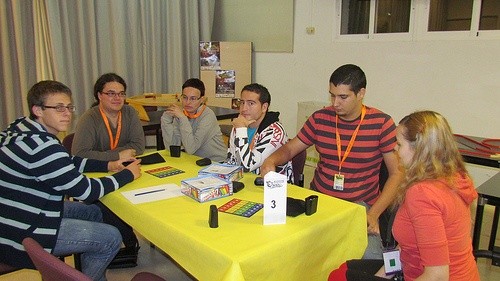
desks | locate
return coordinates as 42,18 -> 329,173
449,134 -> 500,267
76,148 -> 368,281
82,103 -> 241,151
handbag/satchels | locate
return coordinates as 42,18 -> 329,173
96,200 -> 141,269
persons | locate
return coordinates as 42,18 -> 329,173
71,72 -> 146,161
161,78 -> 228,164
260,64 -> 403,259
0,80 -> 142,281
327,110 -> 480,281
227,83 -> 294,184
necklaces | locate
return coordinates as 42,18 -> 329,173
333,104 -> 366,191
182,102 -> 206,119
250,134 -> 257,151
97,105 -> 121,151
382,204 -> 402,274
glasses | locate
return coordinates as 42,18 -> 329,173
102,90 -> 127,97
180,94 -> 201,101
44,104 -> 76,112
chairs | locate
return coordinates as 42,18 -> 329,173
23,237 -> 169,281
292,149 -> 306,186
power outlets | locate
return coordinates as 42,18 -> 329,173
307,27 -> 315,34
